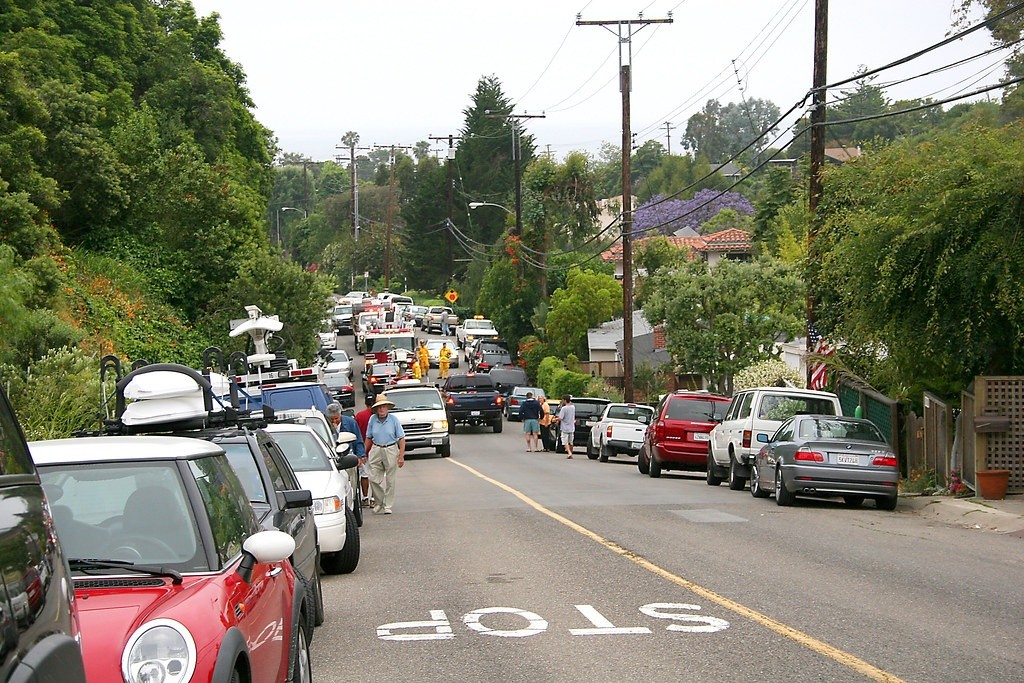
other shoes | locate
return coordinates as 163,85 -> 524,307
526,449 -> 530,452
567,455 -> 573,459
372,505 -> 383,513
370,499 -> 376,507
361,496 -> 369,507
383,507 -> 392,514
535,449 -> 538,452
540,448 -> 550,452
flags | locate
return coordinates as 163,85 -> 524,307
805,321 -> 835,390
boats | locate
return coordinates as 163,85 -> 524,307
100,346 -> 247,436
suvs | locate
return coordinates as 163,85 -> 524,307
380,381 -> 451,459
706,386 -> 843,490
638,390 -> 732,478
549,395 -> 612,451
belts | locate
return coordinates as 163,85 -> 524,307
373,443 -> 395,448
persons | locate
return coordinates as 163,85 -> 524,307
437,343 -> 453,379
324,403 -> 367,498
364,394 -> 406,514
440,308 -> 452,337
537,395 -> 551,452
353,393 -> 376,508
417,339 -> 432,376
519,392 -> 545,453
554,395 -> 576,459
412,357 -> 422,380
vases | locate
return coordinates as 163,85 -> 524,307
976,469 -> 1010,500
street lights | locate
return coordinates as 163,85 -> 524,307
469,203 -> 522,289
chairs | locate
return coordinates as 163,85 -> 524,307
277,438 -> 304,462
123,487 -> 195,560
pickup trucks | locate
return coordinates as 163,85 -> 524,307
442,374 -> 504,434
585,400 -> 654,461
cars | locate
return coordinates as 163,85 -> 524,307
25,435 -> 314,683
324,348 -> 354,379
219,289 -> 561,427
0,384 -> 87,683
750,414 -> 899,511
322,372 -> 356,407
262,423 -> 360,575
180,424 -> 324,625
274,404 -> 364,527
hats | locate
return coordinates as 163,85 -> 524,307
371,394 -> 395,410
